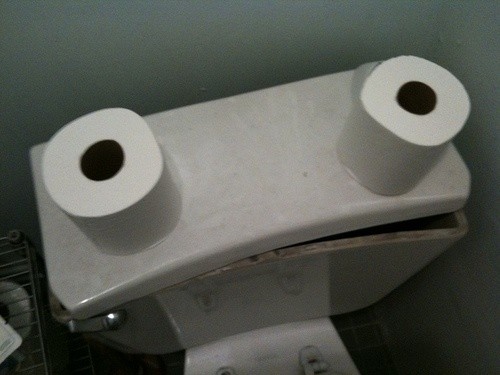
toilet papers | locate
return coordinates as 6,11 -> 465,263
1,281 -> 33,341
334,55 -> 472,196
41,107 -> 183,256
